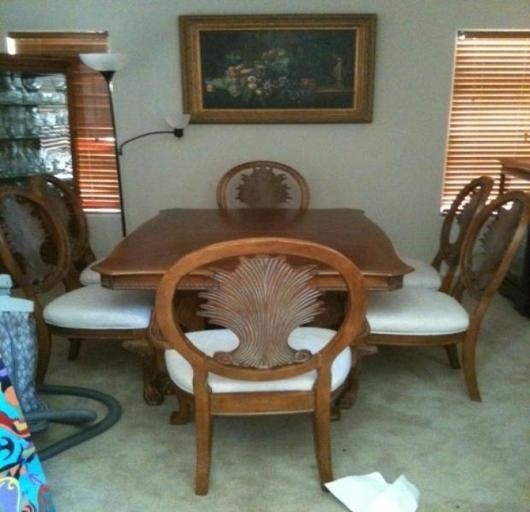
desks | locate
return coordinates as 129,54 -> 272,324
91,207 -> 414,404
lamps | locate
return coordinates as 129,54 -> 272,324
78,52 -> 191,239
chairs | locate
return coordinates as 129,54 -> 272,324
155,237 -> 368,494
339,175 -> 529,411
215,163 -> 310,212
4,172 -> 164,407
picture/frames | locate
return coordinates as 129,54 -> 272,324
178,14 -> 377,125
